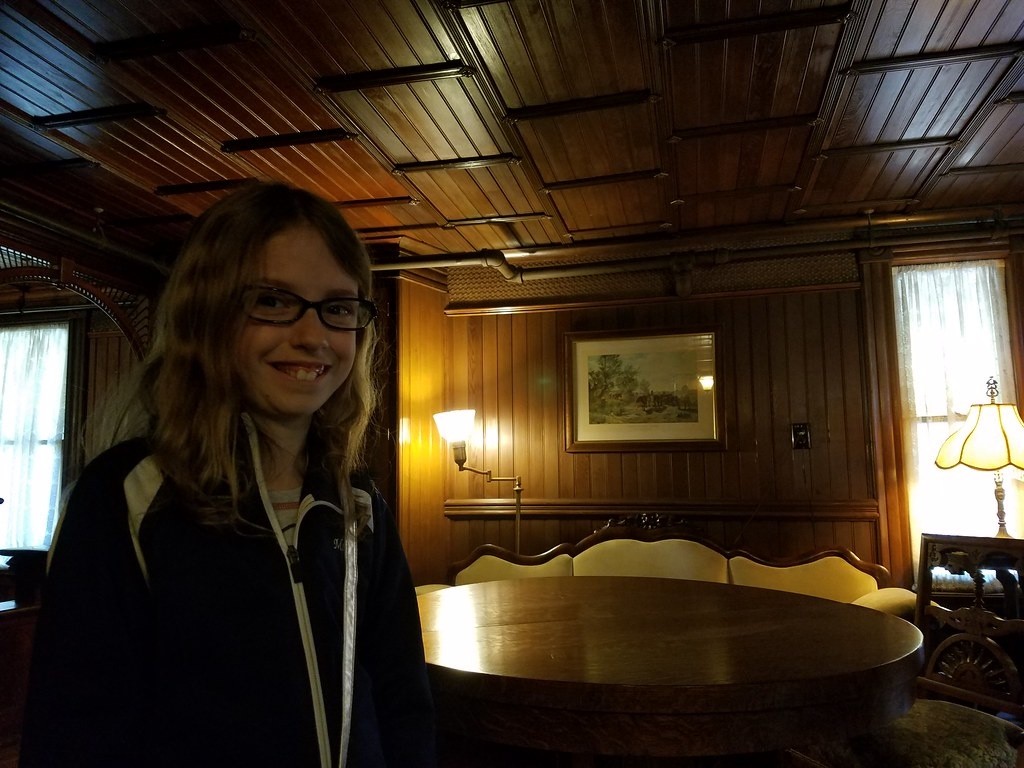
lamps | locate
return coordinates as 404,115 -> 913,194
435,408 -> 522,556
935,378 -> 1024,538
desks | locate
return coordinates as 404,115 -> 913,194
419,578 -> 1024,768
910,534 -> 1024,725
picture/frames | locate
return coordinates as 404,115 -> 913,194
563,327 -> 722,453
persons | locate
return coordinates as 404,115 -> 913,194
16,181 -> 439,768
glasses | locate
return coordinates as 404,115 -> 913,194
240,285 -> 379,331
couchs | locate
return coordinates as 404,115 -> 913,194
451,511 -> 917,615
